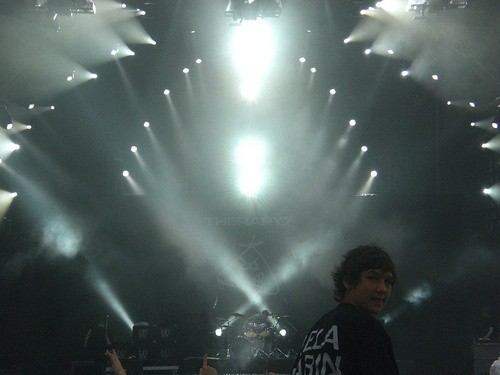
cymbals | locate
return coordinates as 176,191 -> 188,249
231,312 -> 243,319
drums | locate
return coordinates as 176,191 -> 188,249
244,323 -> 257,338
227,336 -> 253,359
257,322 -> 271,337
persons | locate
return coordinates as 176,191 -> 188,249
290,245 -> 400,375
83,306 -> 500,375
